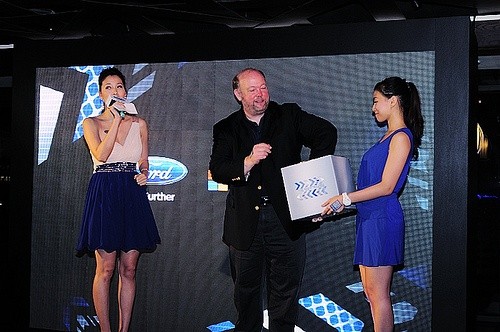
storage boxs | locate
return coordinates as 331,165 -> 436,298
280,155 -> 357,221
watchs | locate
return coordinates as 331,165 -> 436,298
342,192 -> 352,207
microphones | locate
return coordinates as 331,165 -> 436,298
107,95 -> 126,117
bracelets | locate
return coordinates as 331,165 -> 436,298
140,167 -> 149,172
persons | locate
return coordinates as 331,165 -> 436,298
76,68 -> 162,332
311,76 -> 425,332
207,67 -> 339,332
